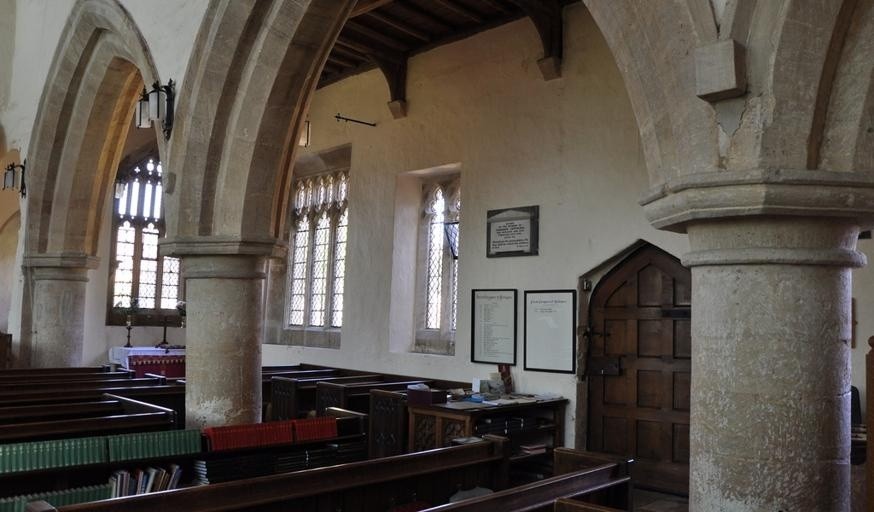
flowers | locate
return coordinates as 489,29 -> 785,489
176,301 -> 186,316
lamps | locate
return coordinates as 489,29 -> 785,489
135,77 -> 176,141
2,158 -> 27,199
299,119 -> 312,146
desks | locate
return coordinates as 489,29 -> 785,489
0,346 -> 636,512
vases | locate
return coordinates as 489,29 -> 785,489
181,316 -> 186,328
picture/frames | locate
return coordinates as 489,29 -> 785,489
470,288 -> 517,366
486,205 -> 540,258
523,289 -> 577,374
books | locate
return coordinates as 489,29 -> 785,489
475,391 -> 564,455
0,414 -> 338,512
